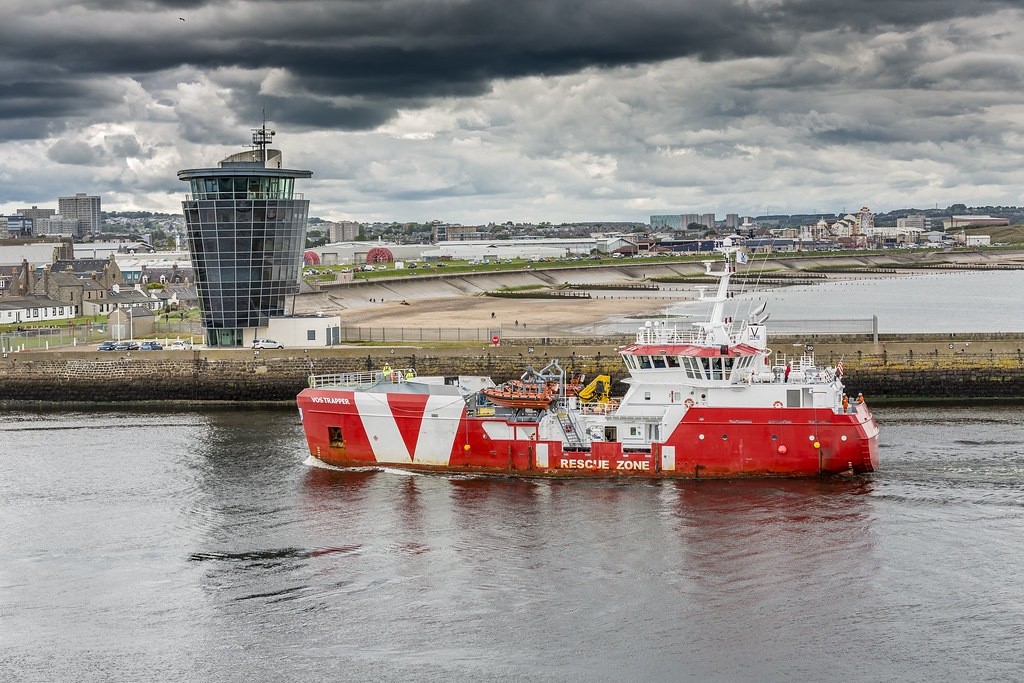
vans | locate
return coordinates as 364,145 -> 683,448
613,253 -> 622,259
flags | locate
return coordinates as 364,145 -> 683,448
838,361 -> 843,379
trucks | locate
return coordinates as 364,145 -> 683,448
395,262 -> 405,269
530,255 -> 540,261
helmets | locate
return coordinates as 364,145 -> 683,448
385,363 -> 388,365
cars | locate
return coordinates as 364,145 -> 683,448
408,263 -> 418,268
550,256 -> 583,262
469,259 -> 478,265
115,341 -> 139,350
524,259 -> 533,263
423,263 -> 430,268
378,265 -> 386,270
437,262 -> 448,267
322,269 -> 332,274
171,341 -> 192,351
309,268 -> 321,275
97,341 -> 117,351
504,260 -> 512,264
586,255 -> 602,260
538,258 -> 550,262
139,340 -> 164,350
364,265 -> 374,271
251,337 -> 285,349
629,251 -> 691,258
494,259 -> 501,265
303,271 -> 312,275
341,267 -> 352,272
481,259 -> 489,265
355,267 -> 362,272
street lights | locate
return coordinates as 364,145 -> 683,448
126,310 -> 132,340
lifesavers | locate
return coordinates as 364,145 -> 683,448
684,399 -> 695,408
609,400 -> 618,409
773,401 -> 784,409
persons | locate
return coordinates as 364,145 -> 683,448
857,393 -> 864,404
784,363 -> 791,383
406,369 -> 413,379
842,392 -> 848,413
383,363 -> 391,380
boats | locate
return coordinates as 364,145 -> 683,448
295,238 -> 886,480
482,356 -> 585,409
